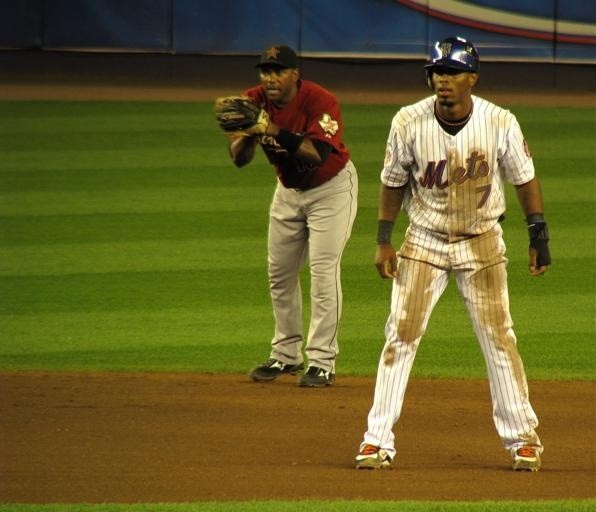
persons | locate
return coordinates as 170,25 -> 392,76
356,35 -> 552,473
214,45 -> 358,388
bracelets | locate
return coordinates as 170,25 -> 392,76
275,129 -> 304,155
527,213 -> 548,240
376,220 -> 394,244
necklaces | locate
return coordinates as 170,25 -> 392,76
435,110 -> 472,126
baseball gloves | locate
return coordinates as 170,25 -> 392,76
215,94 -> 270,136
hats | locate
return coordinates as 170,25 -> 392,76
253,45 -> 297,67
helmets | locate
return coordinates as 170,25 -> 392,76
424,36 -> 479,91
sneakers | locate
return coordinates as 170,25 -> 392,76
354,445 -> 391,469
296,366 -> 334,387
249,358 -> 304,381
511,448 -> 541,472
